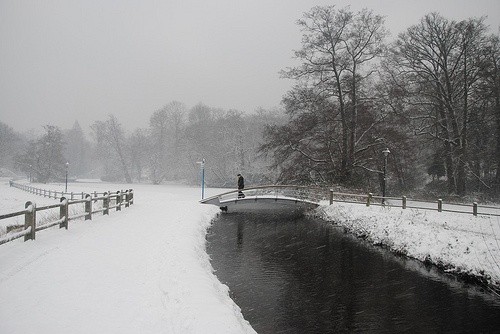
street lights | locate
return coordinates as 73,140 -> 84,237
30,164 -> 32,183
65,162 -> 68,192
382,148 -> 391,204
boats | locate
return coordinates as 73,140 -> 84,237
199,158 -> 322,213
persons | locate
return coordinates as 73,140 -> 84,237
236,173 -> 245,199
